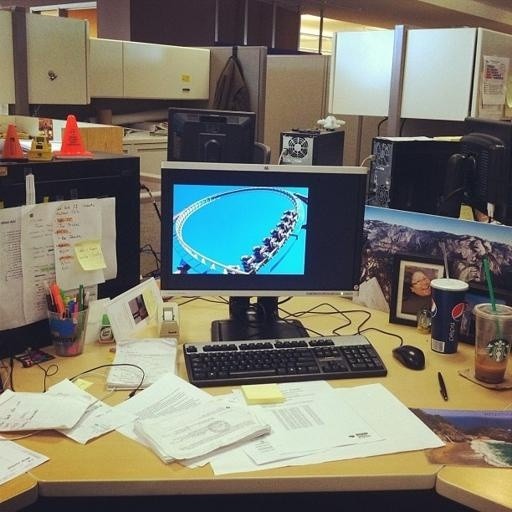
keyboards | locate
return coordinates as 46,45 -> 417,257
184,336 -> 386,386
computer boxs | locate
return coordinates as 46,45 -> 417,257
0,152 -> 140,359
368,136 -> 463,214
280,129 -> 344,166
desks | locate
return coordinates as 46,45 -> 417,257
0,290 -> 512,512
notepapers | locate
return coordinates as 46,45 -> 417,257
241,384 -> 284,405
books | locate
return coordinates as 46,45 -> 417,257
136,395 -> 272,465
105,337 -> 180,391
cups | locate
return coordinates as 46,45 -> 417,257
47,308 -> 90,356
95,108 -> 113,125
427,278 -> 471,352
473,303 -> 512,383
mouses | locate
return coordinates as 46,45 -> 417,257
392,345 -> 425,370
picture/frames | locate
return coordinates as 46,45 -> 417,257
388,253 -> 446,328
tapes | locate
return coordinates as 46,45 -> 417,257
164,311 -> 172,321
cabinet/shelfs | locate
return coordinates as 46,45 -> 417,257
121,136 -> 168,176
0,13 -> 90,106
89,36 -> 211,101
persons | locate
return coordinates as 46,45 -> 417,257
402,270 -> 431,316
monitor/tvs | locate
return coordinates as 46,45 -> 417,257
167,107 -> 256,164
441,118 -> 512,226
159,161 -> 367,340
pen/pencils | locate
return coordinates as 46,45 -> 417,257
438,372 -> 448,400
42,280 -> 90,342
